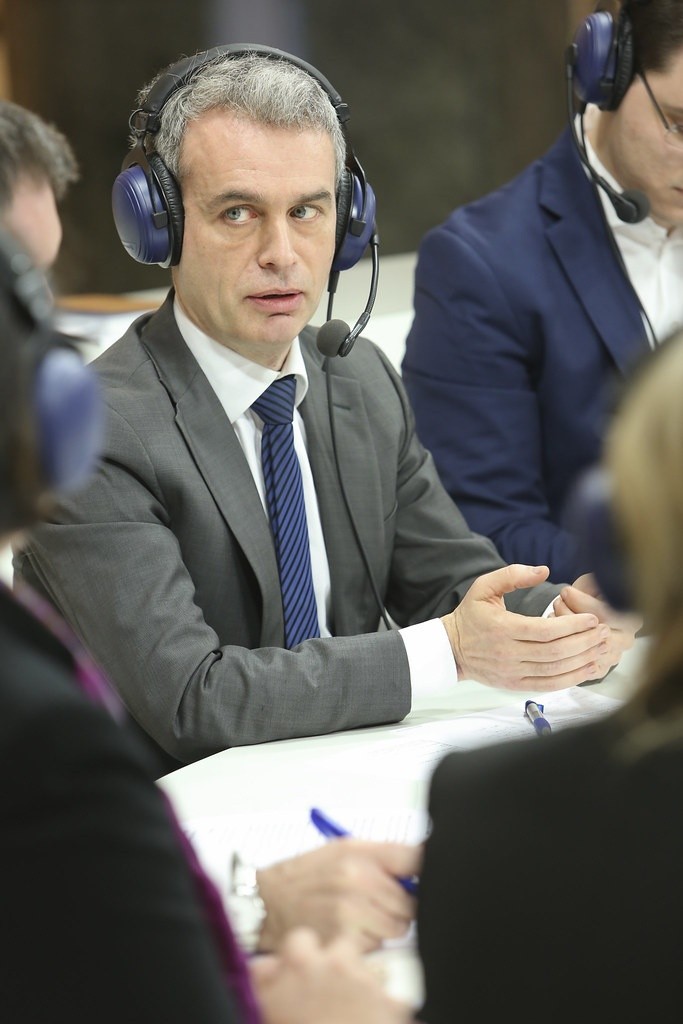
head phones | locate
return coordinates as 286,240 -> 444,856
111,43 -> 376,271
1,221 -> 107,495
565,0 -> 635,113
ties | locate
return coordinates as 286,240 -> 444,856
12,575 -> 266,1024
250,378 -> 321,650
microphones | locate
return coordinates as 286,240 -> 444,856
565,77 -> 651,223
316,245 -> 379,357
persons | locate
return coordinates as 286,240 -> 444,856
14,42 -> 644,782
411,324 -> 683,1024
0,97 -> 426,1024
399,0 -> 683,601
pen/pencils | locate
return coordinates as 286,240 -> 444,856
308,807 -> 420,898
525,699 -> 551,737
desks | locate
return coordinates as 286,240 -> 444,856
153,637 -> 650,1008
